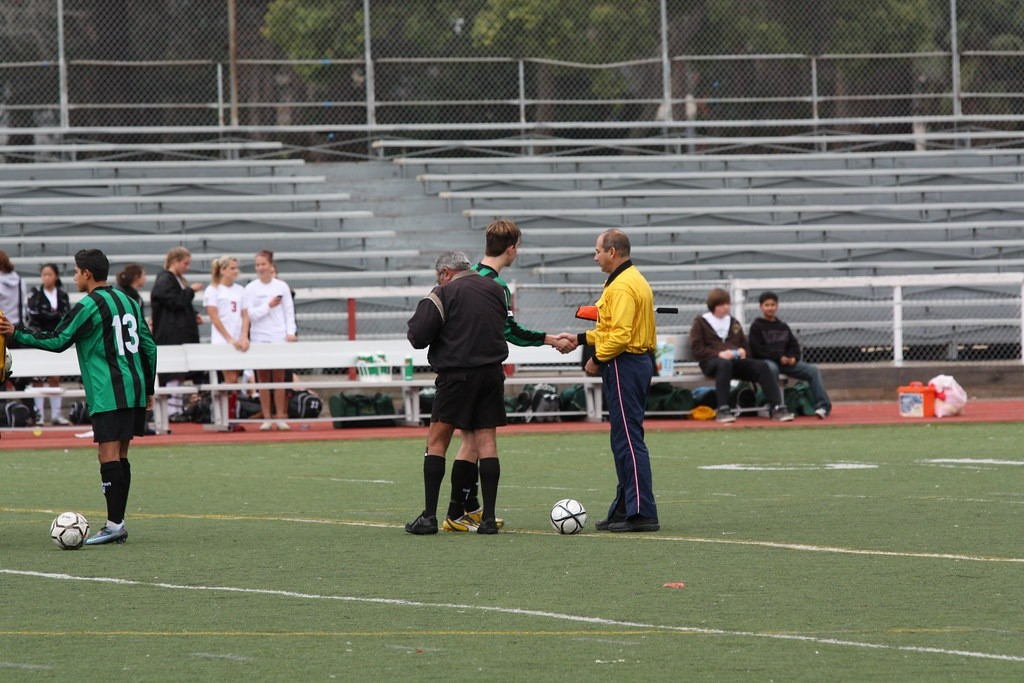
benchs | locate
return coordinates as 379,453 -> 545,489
0,130 -> 1024,347
0,386 -> 199,435
195,368 -> 797,433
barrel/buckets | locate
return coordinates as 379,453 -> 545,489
897,382 -> 935,418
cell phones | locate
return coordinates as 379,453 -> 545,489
277,295 -> 282,298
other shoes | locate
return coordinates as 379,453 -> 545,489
771,405 -> 795,422
51,415 -> 69,425
716,408 -> 736,422
260,421 -> 272,429
814,408 -> 826,419
84,523 -> 128,544
273,419 -> 290,431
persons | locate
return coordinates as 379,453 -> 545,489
689,288 -> 793,423
0,249 -> 157,546
443,217 -> 576,532
552,229 -> 660,533
406,250 -> 509,535
749,292 -> 831,419
115,247 -> 204,436
202,251 -> 298,433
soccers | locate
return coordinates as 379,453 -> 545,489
49,511 -> 90,550
550,499 -> 588,534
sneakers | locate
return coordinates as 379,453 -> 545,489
477,517 -> 498,534
442,514 -> 480,532
596,515 -> 628,530
404,511 -> 438,534
608,516 -> 660,532
460,510 -> 504,528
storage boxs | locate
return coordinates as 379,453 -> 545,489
897,381 -> 936,418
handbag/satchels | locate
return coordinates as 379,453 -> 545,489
2,382 -> 827,420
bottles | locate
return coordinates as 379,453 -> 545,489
405,354 -> 413,380
358,352 -> 386,376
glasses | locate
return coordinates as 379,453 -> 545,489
435,271 -> 444,286
